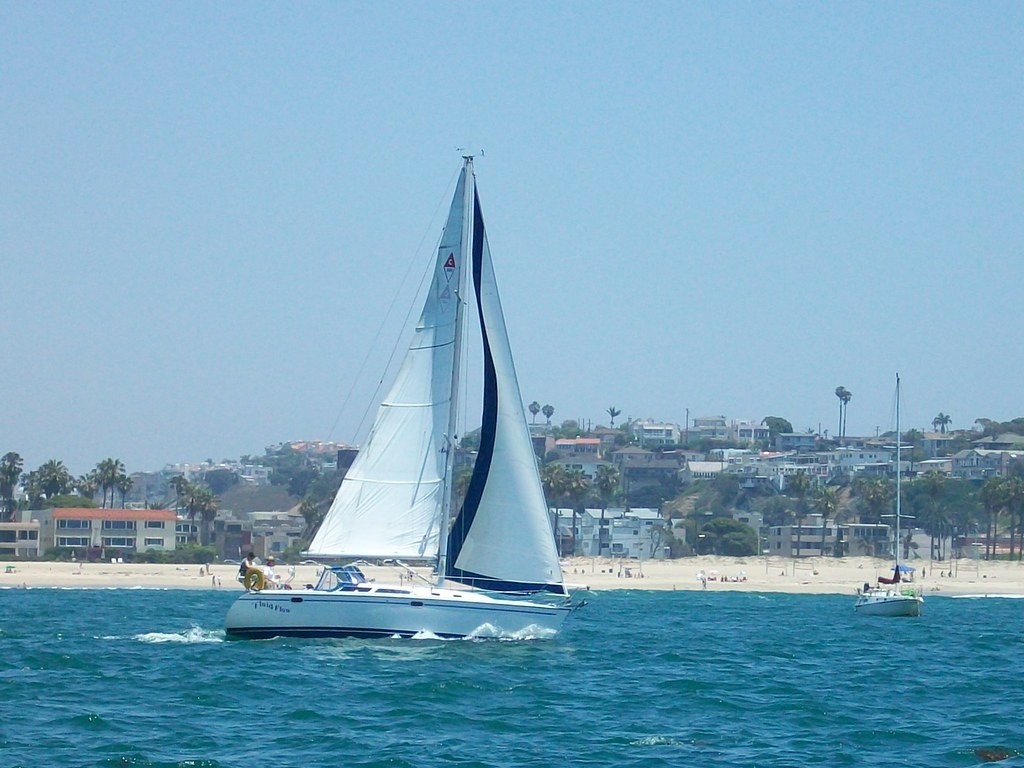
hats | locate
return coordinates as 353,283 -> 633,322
247,552 -> 256,558
266,555 -> 275,561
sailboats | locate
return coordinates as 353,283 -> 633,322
853,374 -> 925,618
221,143 -> 594,643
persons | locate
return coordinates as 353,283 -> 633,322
200,561 -> 222,588
241,551 -> 282,588
